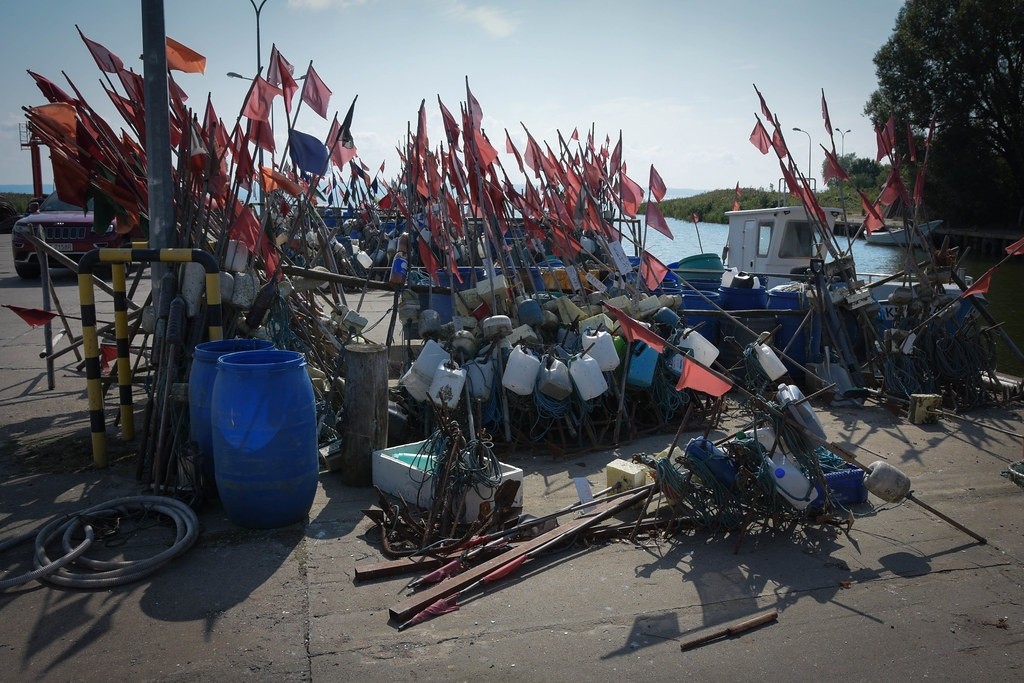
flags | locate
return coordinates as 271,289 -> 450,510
2,34 -> 1024,399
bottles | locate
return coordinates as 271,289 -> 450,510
355,251 -> 375,267
579,235 -> 594,255
393,258 -> 825,510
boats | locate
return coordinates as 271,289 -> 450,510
863,220 -> 944,247
717,176 -> 991,342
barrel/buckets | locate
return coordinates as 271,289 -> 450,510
187,338 -> 282,454
680,287 -> 717,360
718,284 -> 774,388
766,290 -> 822,384
210,350 -> 320,530
402,234 -> 727,325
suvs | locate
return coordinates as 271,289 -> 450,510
11,190 -> 152,280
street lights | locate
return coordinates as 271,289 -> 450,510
792,127 -> 811,189
836,128 -> 851,221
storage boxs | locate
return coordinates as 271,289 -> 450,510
746,428 -> 868,511
371,440 -> 523,523
